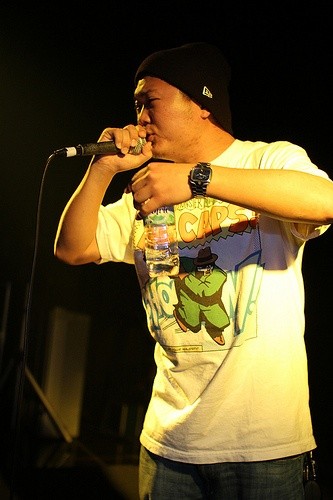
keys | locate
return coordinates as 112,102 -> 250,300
303,449 -> 319,486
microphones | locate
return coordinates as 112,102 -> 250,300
55,136 -> 147,159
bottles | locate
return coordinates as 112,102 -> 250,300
142,196 -> 180,277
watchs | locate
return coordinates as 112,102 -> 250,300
189,161 -> 213,199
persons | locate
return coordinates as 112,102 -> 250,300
53,41 -> 332,500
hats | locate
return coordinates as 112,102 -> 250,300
134,40 -> 232,133
